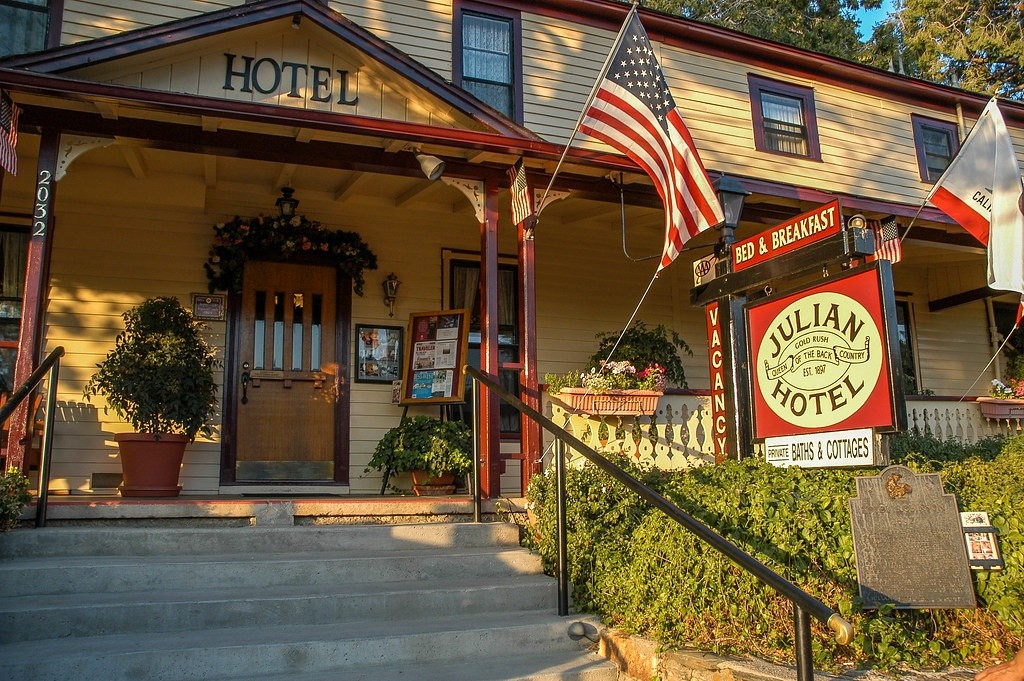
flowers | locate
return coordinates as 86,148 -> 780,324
988,377 -> 1024,400
545,359 -> 669,396
203,211 -> 378,299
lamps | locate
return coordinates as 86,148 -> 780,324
413,145 -> 445,180
382,271 -> 403,319
620,172 -> 751,261
275,185 -> 300,218
848,210 -> 867,230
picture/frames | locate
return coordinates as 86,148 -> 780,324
355,323 -> 403,384
190,292 -> 226,321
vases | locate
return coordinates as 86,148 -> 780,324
558,387 -> 664,416
975,396 -> 1024,430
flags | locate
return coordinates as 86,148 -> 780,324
925,97 -> 1024,329
869,214 -> 901,264
579,6 -> 724,275
0,88 -> 21,176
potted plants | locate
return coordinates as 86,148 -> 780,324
82,295 -> 226,497
359,414 -> 474,496
